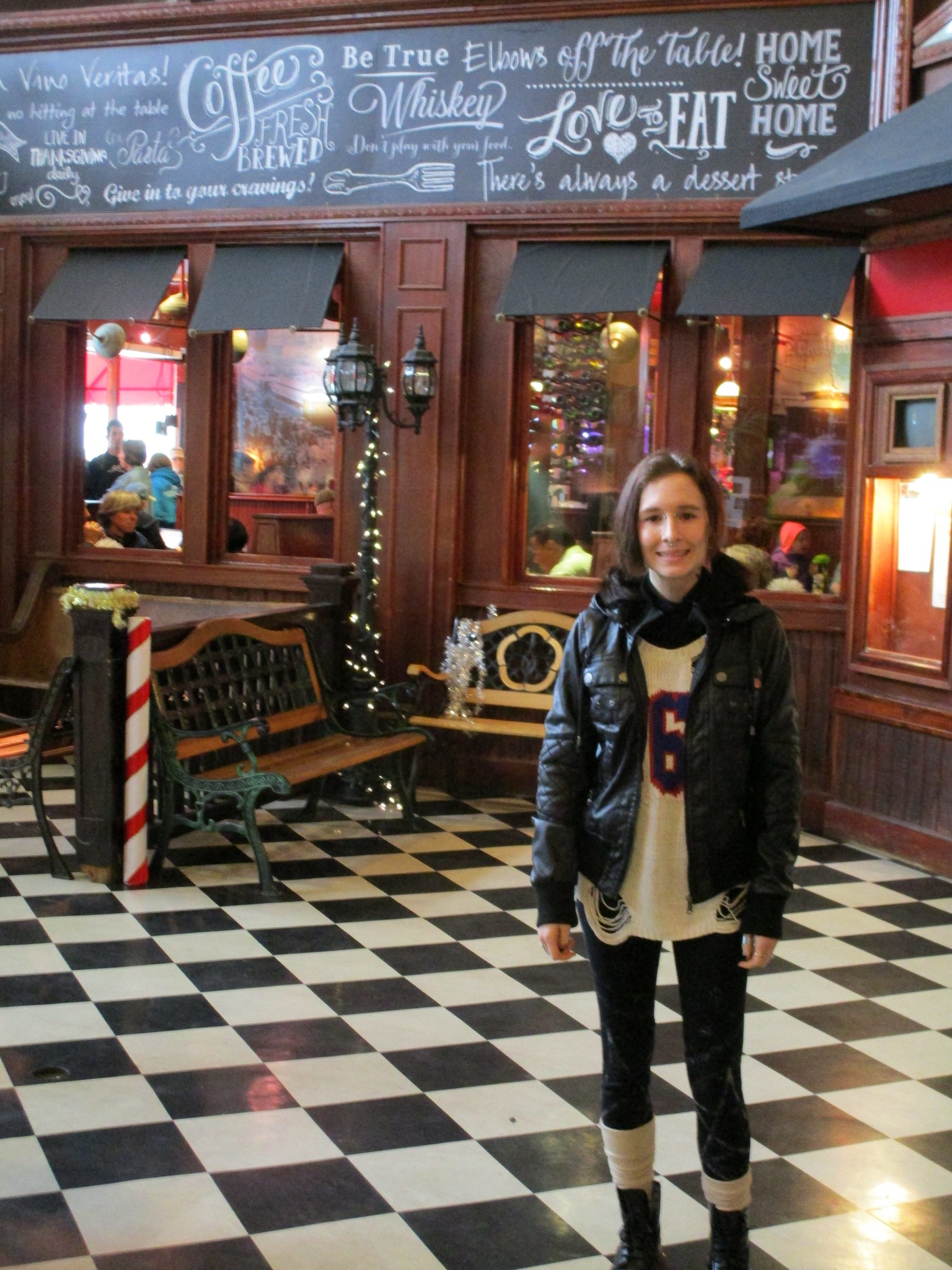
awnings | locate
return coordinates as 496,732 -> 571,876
676,237 -> 864,316
740,84 -> 952,236
493,240 -> 672,313
29,243 -> 186,324
189,238 -> 345,336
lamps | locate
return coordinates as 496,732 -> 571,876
160,259 -> 190,322
85,315 -> 126,357
800,329 -> 850,409
598,312 -> 638,364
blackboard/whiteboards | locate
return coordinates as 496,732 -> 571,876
0,0 -> 913,240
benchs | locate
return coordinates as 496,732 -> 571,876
145,621 -> 433,896
0,655 -> 79,882
407,610 -> 585,808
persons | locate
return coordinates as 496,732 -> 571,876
231,448 -> 328,498
530,450 -> 805,1270
531,519 -> 593,577
724,517 -> 774,590
82,419 -> 185,550
226,515 -> 250,554
314,489 -> 335,518
770,522 -> 814,586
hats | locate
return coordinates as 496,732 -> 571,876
127,482 -> 156,502
171,446 -> 185,460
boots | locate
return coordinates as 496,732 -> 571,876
611,1180 -> 661,1270
708,1202 -> 750,1270
